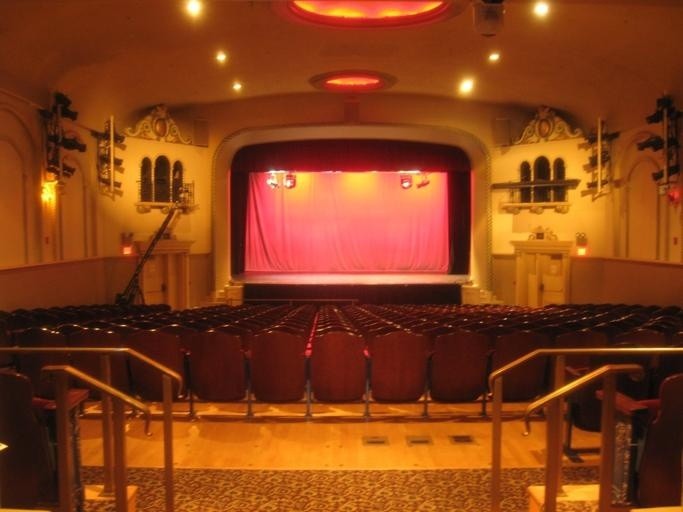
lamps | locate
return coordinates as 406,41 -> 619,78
577,235 -> 587,256
121,233 -> 133,255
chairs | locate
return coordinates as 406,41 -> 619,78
595,376 -> 683,508
0,369 -> 58,509
